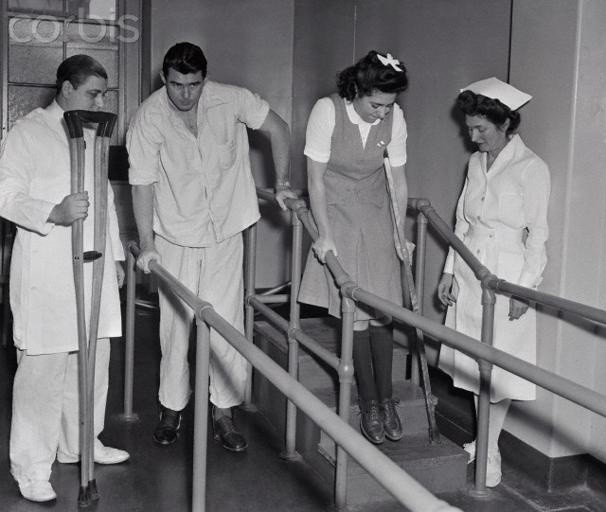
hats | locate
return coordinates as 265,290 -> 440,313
460,76 -> 533,112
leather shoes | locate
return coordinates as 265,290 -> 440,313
154,409 -> 182,445
359,398 -> 404,444
213,415 -> 248,453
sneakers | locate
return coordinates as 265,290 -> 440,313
57,444 -> 130,464
463,441 -> 477,464
9,467 -> 57,503
486,448 -> 502,487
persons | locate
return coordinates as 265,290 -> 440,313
0,55 -> 131,503
438,77 -> 551,488
126,42 -> 299,451
297,50 -> 417,444
